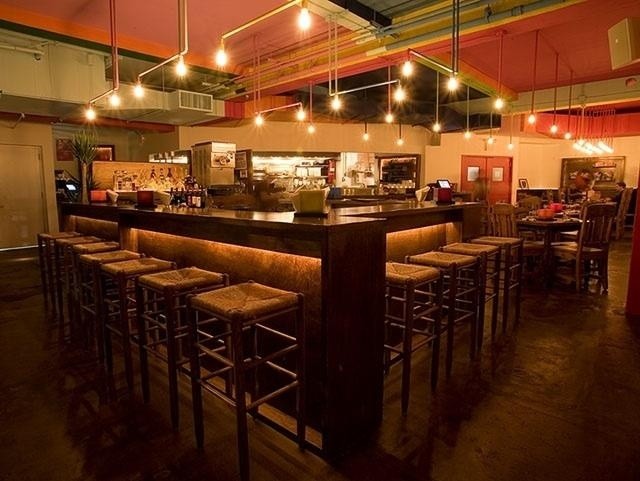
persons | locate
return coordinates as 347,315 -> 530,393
608,181 -> 637,225
575,169 -> 595,193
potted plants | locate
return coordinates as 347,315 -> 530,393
62,131 -> 100,206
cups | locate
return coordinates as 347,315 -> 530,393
562,209 -> 571,222
529,210 -> 537,221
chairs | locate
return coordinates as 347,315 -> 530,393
488,187 -> 633,303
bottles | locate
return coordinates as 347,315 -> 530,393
112,169 -> 140,192
149,167 -> 200,189
170,186 -> 205,209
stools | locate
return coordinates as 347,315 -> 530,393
37,230 -> 120,337
134,266 -> 233,451
78,248 -> 144,375
101,257 -> 182,410
383,236 -> 534,418
185,280 -> 309,480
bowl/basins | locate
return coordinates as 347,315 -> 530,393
550,203 -> 562,212
290,187 -> 330,214
538,208 -> 555,218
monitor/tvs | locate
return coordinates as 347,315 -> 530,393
66,184 -> 76,190
438,180 -> 449,187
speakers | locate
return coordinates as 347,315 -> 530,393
607,18 -> 640,70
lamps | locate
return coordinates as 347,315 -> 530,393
82,0 -> 619,162
